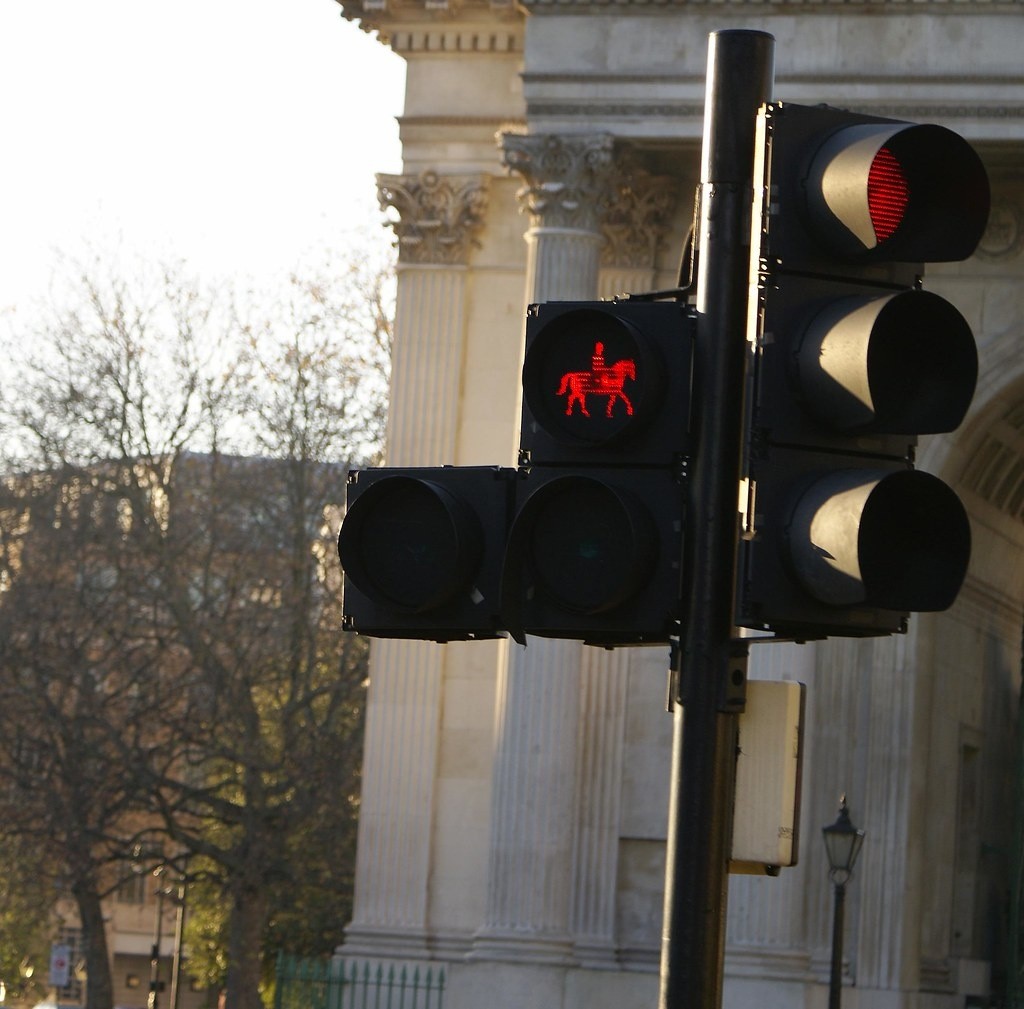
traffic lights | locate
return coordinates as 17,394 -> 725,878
333,297 -> 699,647
724,94 -> 994,639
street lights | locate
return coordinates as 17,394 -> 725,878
819,793 -> 867,1009
